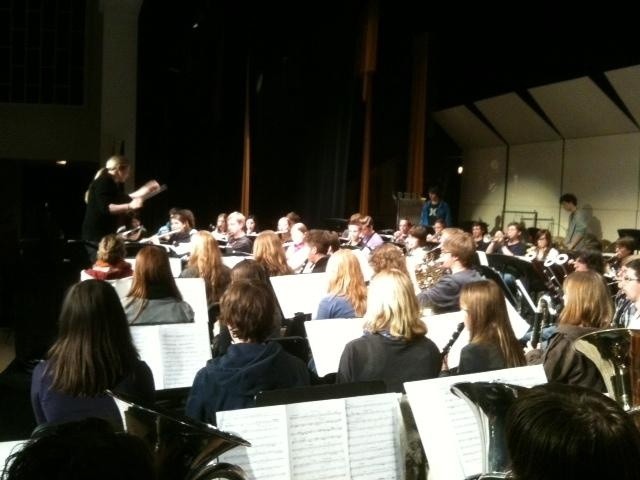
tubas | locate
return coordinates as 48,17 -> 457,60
572,327 -> 640,431
447,379 -> 532,478
103,383 -> 256,480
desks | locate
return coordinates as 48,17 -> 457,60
125,237 -> 255,258
149,369 -> 456,419
397,198 -> 425,230
15,240 -> 83,365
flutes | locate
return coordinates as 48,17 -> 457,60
438,319 -> 465,367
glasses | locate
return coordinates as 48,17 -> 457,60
621,277 -> 639,283
441,250 -> 457,255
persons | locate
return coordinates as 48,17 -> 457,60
279,211 -> 301,243
470,222 -> 488,249
82,234 -> 131,285
523,274 -> 633,392
281,222 -> 311,274
611,237 -> 637,267
507,384 -> 638,479
211,260 -> 286,357
212,213 -> 231,244
120,249 -> 193,325
341,223 -> 363,248
176,230 -> 232,311
221,213 -> 253,258
243,218 -> 255,239
454,281 -> 525,373
249,231 -> 294,276
495,221 -> 532,260
612,260 -> 639,331
86,155 -> 143,258
29,281 -> 155,428
116,211 -> 146,243
420,185 -> 452,228
405,225 -> 438,294
170,212 -> 199,252
558,192 -> 593,249
523,230 -> 558,265
370,244 -> 408,277
575,252 -> 603,277
318,251 -> 367,321
336,269 -> 443,391
419,228 -> 495,315
186,281 -> 311,423
393,218 -> 410,245
299,230 -> 330,274
359,217 -> 380,247
1,416 -> 158,479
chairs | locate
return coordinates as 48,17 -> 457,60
286,314 -> 310,336
268,336 -> 308,364
254,380 -> 388,406
